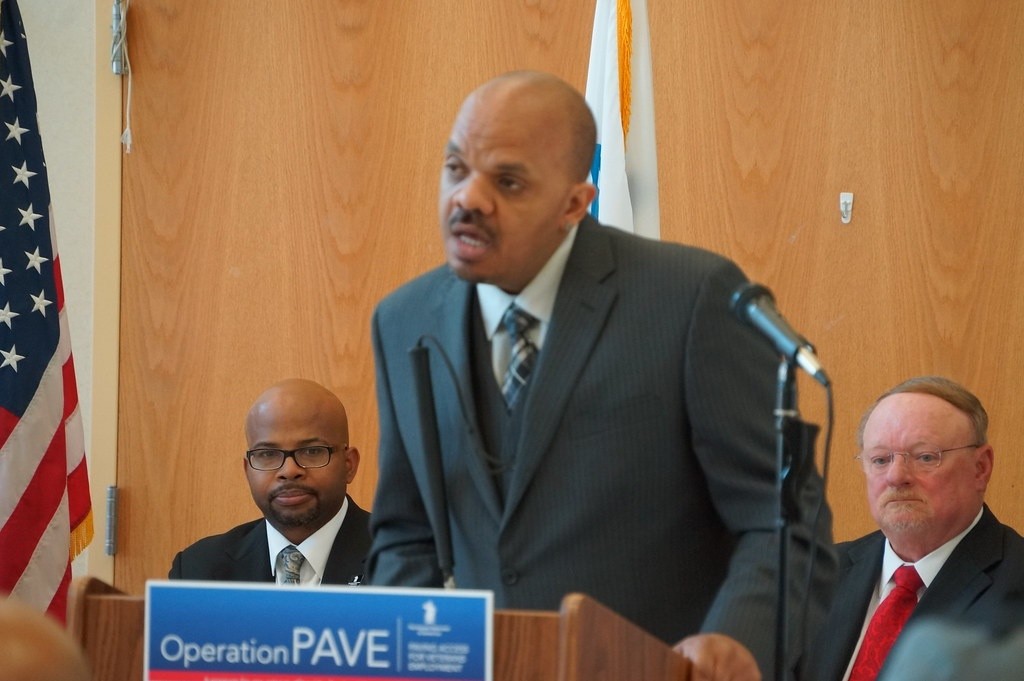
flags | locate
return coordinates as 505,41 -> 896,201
0,0 -> 94,633
584,0 -> 663,242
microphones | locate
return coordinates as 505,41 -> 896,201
728,284 -> 829,391
409,349 -> 455,570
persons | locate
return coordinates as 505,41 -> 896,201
361,70 -> 841,680
832,376 -> 1024,680
166,379 -> 374,586
0,598 -> 91,681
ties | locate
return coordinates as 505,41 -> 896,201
847,566 -> 926,681
500,304 -> 541,415
277,547 -> 306,584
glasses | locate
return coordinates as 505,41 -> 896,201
246,443 -> 350,471
852,443 -> 979,475
